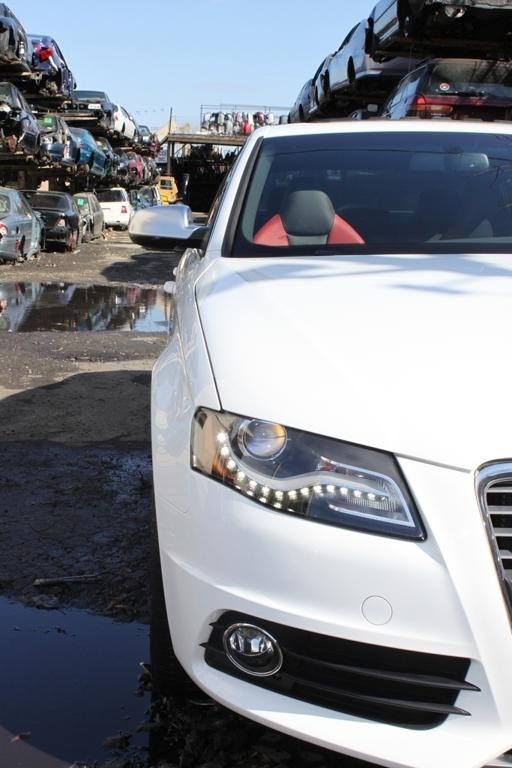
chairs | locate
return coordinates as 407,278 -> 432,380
253,188 -> 367,246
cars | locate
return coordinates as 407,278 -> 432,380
287,1 -> 512,121
128,122 -> 512,759
